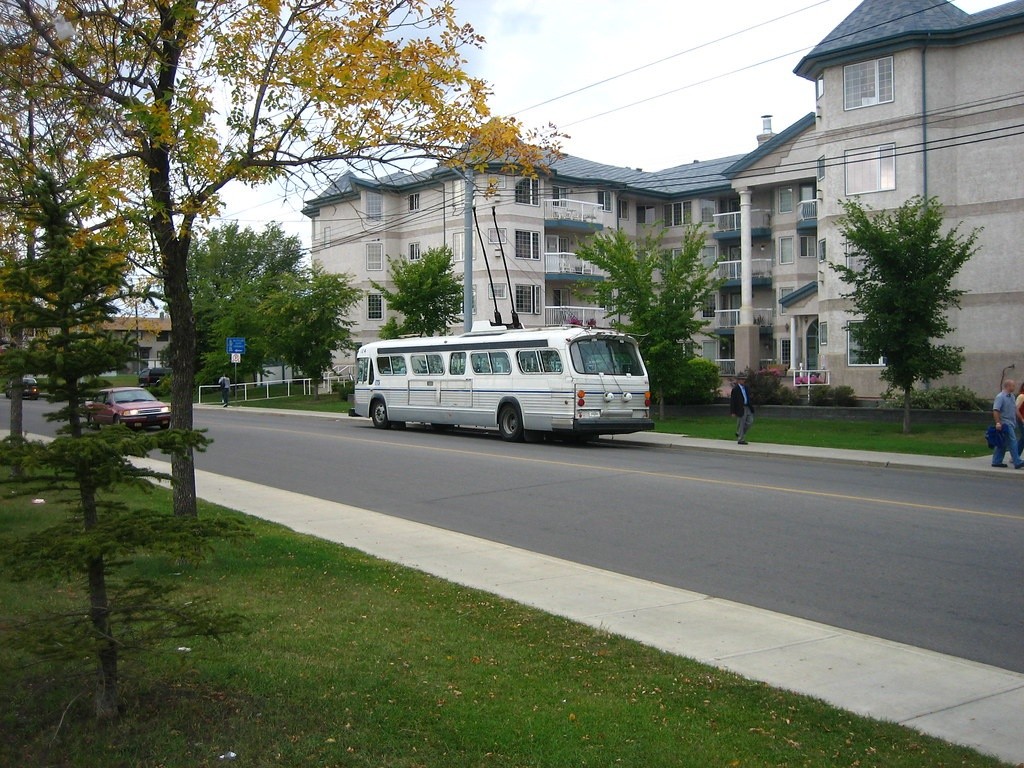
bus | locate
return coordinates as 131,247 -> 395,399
349,324 -> 655,443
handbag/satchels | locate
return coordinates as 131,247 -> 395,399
221,376 -> 225,389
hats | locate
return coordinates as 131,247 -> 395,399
738,373 -> 747,378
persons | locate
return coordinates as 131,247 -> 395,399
991,379 -> 1024,469
218,373 -> 230,407
731,372 -> 755,445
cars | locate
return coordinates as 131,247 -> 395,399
138,367 -> 172,387
5,377 -> 39,400
86,387 -> 173,432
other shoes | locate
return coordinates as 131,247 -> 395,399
1015,461 -> 1024,469
736,432 -> 739,437
992,463 -> 1007,467
738,442 -> 748,444
223,404 -> 227,407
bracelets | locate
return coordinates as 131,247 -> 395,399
995,422 -> 1001,424
